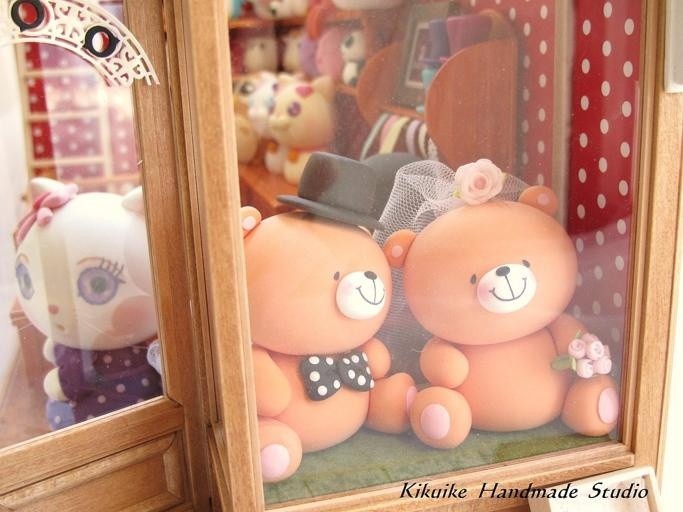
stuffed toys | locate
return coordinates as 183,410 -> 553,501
9,0 -> 625,485
